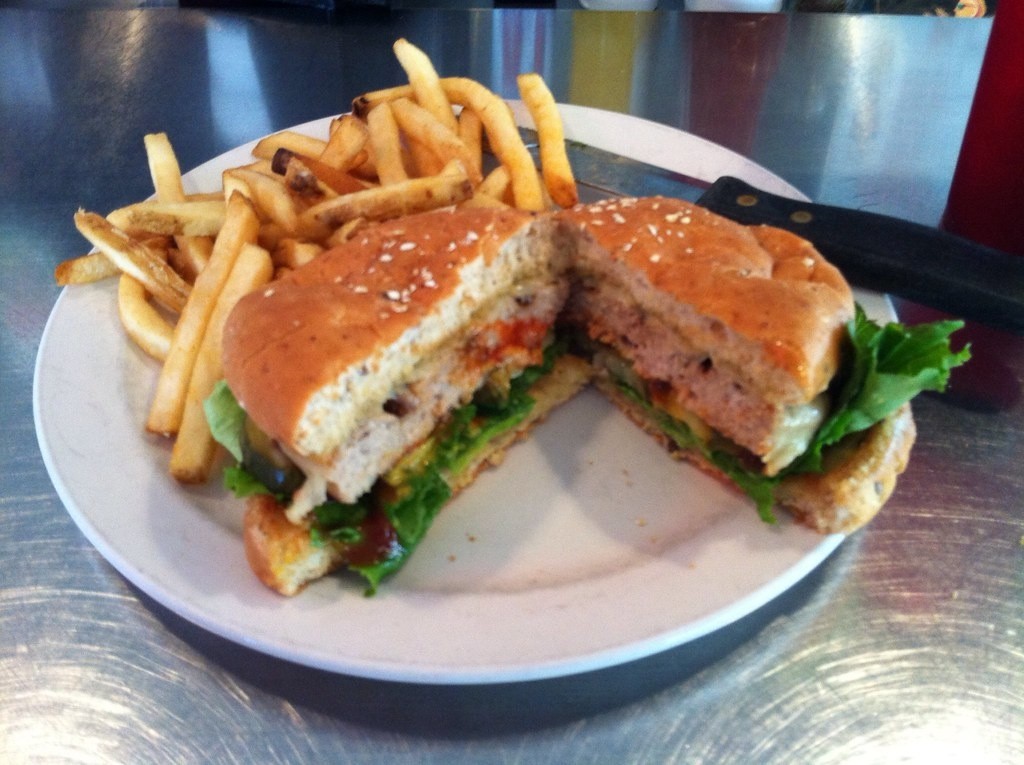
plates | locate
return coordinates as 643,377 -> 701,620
33,98 -> 899,684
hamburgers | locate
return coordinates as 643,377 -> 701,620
203,204 -> 601,595
556,193 -> 971,536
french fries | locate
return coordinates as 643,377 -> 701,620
53,39 -> 579,485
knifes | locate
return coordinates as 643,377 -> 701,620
478,121 -> 1024,335
